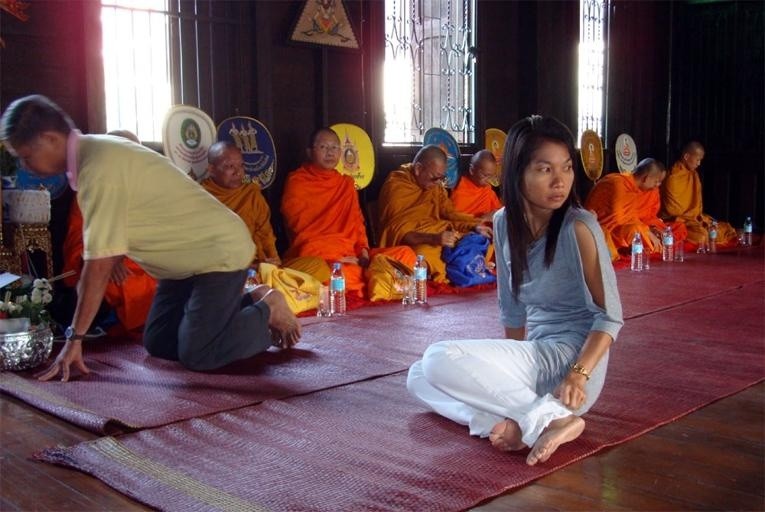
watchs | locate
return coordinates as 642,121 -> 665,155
571,363 -> 590,381
64,327 -> 85,340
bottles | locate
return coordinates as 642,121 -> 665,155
709,222 -> 713,251
245,271 -> 256,292
631,232 -> 641,271
744,217 -> 751,245
330,263 -> 346,315
415,255 -> 426,303
662,228 -> 673,260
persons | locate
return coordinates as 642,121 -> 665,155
638,163 -> 688,245
63,130 -> 158,329
449,150 -> 503,233
623,138 -> 632,155
246,121 -> 258,152
662,143 -> 735,244
230,122 -> 243,151
585,158 -> 658,256
239,123 -> 250,152
376,144 -> 494,283
405,114 -> 625,467
198,141 -> 329,283
280,125 -> 417,297
588,143 -> 595,163
2,94 -> 302,383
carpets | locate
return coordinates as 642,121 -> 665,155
0,232 -> 764,435
28,280 -> 765,512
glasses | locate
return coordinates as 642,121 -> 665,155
312,144 -> 340,152
424,169 -> 446,181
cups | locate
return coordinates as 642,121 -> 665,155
402,274 -> 418,304
696,236 -> 705,254
674,241 -> 684,261
642,248 -> 650,270
316,287 -> 332,317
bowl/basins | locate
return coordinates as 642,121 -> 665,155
0,317 -> 54,372
